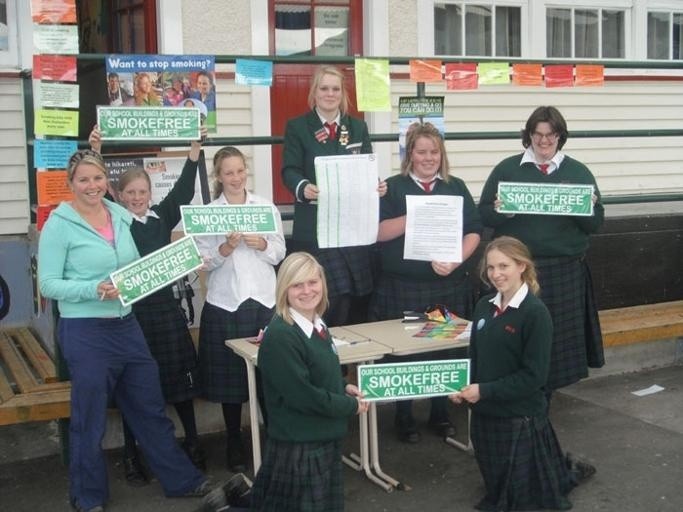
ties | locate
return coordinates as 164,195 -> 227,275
538,161 -> 552,175
323,120 -> 340,142
418,179 -> 437,194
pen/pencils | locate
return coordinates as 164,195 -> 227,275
349,338 -> 372,346
101,289 -> 106,301
378,176 -> 381,184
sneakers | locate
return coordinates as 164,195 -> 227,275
428,412 -> 458,437
181,472 -> 254,512
395,415 -> 421,444
69,492 -> 104,512
565,451 -> 597,480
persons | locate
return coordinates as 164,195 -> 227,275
195,249 -> 373,512
279,64 -> 388,325
34,148 -> 218,512
446,234 -> 596,511
368,121 -> 483,442
88,124 -> 208,488
478,105 -> 607,389
106,71 -> 215,106
199,143 -> 287,474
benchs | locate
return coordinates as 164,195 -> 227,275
0,299 -> 683,425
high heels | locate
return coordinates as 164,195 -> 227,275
183,441 -> 206,469
227,442 -> 247,474
122,455 -> 146,487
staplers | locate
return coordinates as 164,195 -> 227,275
401,311 -> 428,323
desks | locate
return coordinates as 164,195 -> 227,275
223,327 -> 392,493
343,317 -> 473,490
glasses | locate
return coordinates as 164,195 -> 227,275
530,130 -> 560,141
